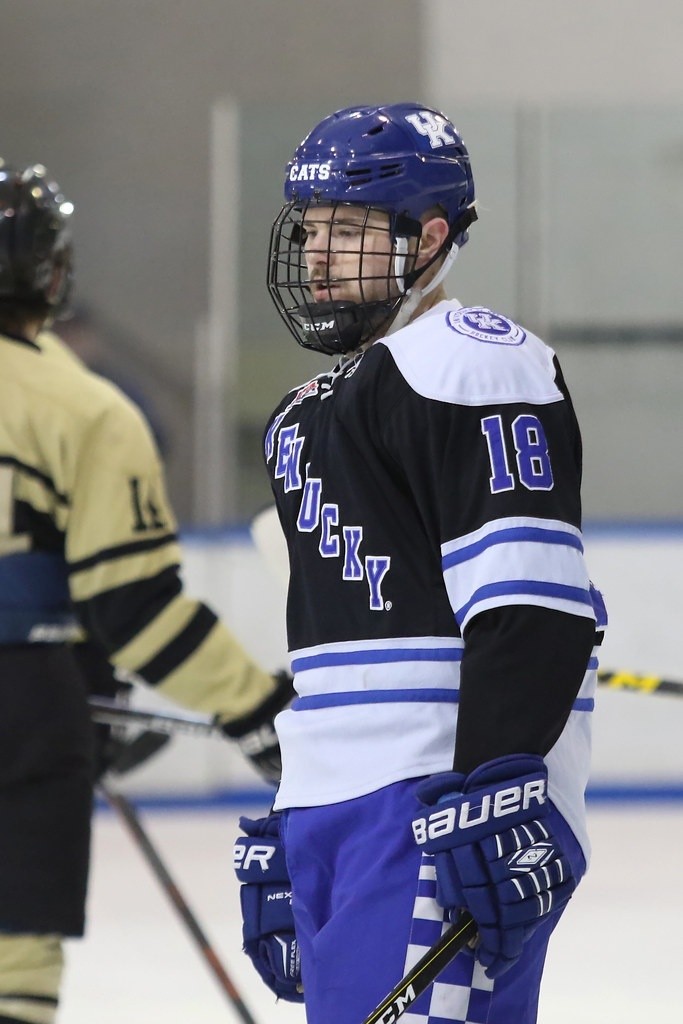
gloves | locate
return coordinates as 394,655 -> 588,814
211,670 -> 299,788
415,754 -> 576,979
234,813 -> 305,1003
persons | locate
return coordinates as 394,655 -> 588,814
234,104 -> 610,1024
0,157 -> 298,1024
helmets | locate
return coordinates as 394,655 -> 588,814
266,102 -> 478,357
0,157 -> 75,327
